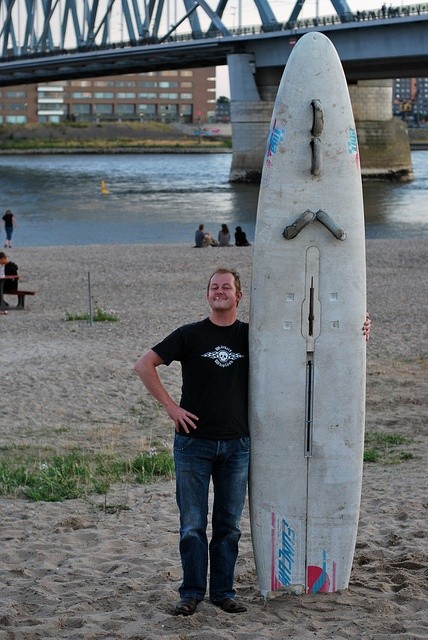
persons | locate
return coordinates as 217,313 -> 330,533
134,268 -> 371,616
232,227 -> 248,249
218,222 -> 233,247
2,207 -> 16,248
194,221 -> 215,247
1,249 -> 34,312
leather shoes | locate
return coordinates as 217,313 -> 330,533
212,597 -> 247,613
175,597 -> 198,615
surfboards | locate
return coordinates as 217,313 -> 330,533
248,32 -> 368,600
